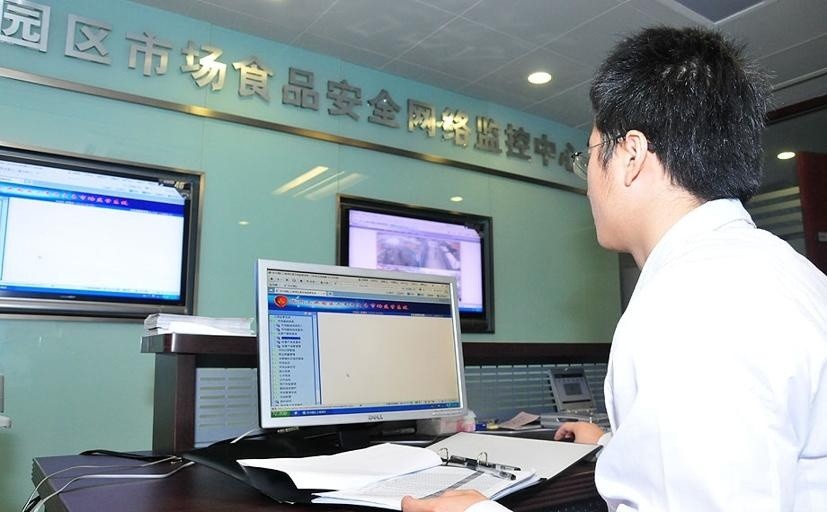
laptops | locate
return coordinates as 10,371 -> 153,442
540,367 -> 610,429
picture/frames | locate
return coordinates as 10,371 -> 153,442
547,366 -> 596,413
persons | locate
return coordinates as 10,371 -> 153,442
402,26 -> 827,512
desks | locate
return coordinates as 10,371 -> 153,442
32,440 -> 625,511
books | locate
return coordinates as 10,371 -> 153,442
143,313 -> 258,335
496,412 -> 542,430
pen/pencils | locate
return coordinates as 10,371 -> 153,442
463,462 -> 516,480
450,455 -> 521,470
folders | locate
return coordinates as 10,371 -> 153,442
298,431 -> 605,512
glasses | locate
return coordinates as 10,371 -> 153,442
573,133 -> 625,182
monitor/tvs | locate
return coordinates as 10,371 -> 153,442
341,203 -> 486,318
1,148 -> 191,308
257,258 -> 468,445
